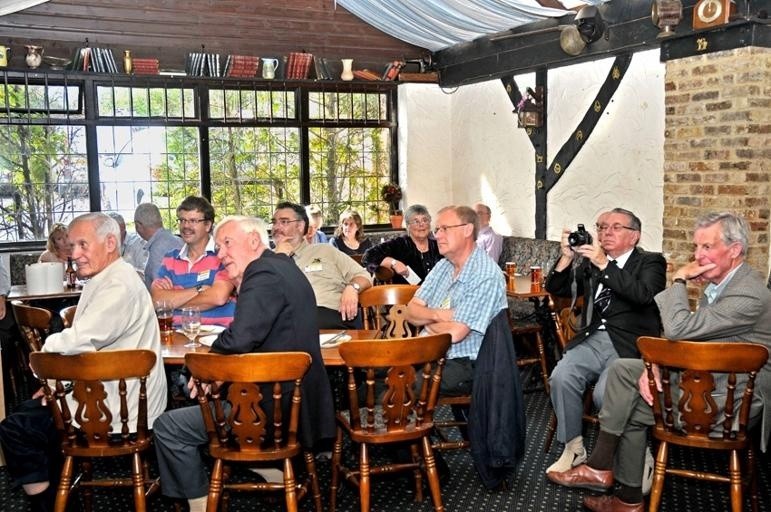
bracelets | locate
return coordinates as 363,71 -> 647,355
672,276 -> 686,285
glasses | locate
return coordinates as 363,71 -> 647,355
601,224 -> 637,235
409,217 -> 432,226
174,217 -> 210,226
433,223 -> 470,234
266,218 -> 304,228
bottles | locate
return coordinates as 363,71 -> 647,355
123,50 -> 133,75
65,256 -> 76,289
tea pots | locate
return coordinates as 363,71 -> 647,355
260,57 -> 280,80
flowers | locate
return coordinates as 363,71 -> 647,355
378,183 -> 404,216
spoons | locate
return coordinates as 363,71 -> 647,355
329,332 -> 346,343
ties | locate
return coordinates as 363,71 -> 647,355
593,259 -> 617,312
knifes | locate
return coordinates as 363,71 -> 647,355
322,330 -> 346,345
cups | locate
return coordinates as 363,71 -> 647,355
542,261 -> 554,284
531,282 -> 541,294
507,277 -> 516,291
0,46 -> 8,67
507,262 -> 516,280
529,266 -> 542,285
154,299 -> 174,335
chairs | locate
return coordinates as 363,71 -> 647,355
339,250 -> 399,287
541,290 -> 601,453
29,347 -> 163,509
0,281 -> 79,402
634,328 -> 771,512
329,331 -> 456,509
357,282 -> 432,342
184,349 -> 324,510
435,304 -> 517,453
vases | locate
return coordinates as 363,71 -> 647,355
340,57 -> 354,80
389,215 -> 404,229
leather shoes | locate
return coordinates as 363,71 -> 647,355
582,493 -> 645,512
546,462 -> 614,492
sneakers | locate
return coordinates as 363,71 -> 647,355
545,448 -> 588,476
640,456 -> 655,498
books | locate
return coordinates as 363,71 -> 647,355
71,44 -> 439,83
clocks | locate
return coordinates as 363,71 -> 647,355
689,0 -> 730,33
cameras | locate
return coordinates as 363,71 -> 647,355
568,224 -> 592,247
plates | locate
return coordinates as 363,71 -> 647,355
197,324 -> 227,336
199,334 -> 218,347
319,332 -> 352,349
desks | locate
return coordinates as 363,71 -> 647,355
501,272 -> 557,399
154,326 -> 386,370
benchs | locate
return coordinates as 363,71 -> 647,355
476,235 -> 566,336
320,228 -> 412,261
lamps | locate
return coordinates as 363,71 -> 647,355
512,84 -> 547,144
573,5 -> 608,45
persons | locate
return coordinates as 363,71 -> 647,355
475,205 -> 502,264
134,204 -> 185,292
38,223 -> 78,281
388,205 -> 510,495
151,194 -> 237,329
0,211 -> 168,510
1,265 -> 11,320
555,211 -> 613,362
153,214 -> 340,510
110,213 -> 147,271
544,207 -> 667,497
548,209 -> 771,511
271,205 -> 444,329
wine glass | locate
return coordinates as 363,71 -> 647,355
180,304 -> 202,348
